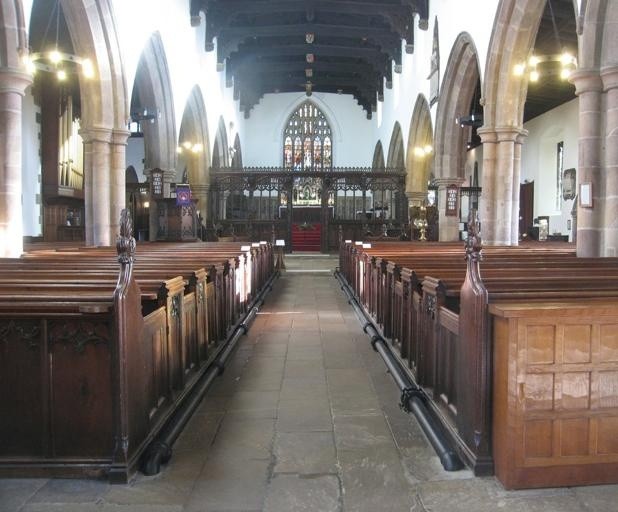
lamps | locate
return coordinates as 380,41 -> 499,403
132,83 -> 161,123
512,0 -> 578,82
177,126 -> 202,154
455,84 -> 483,129
413,124 -> 432,158
20,0 -> 95,83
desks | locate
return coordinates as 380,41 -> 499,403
59,225 -> 84,241
547,236 -> 568,242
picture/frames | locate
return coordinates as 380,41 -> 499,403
577,182 -> 593,208
